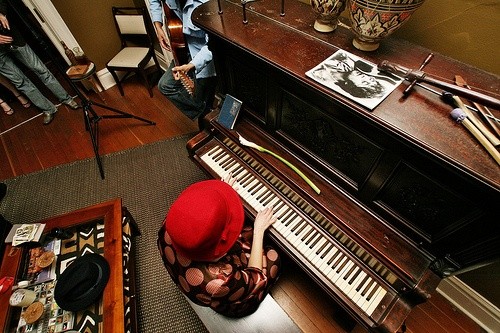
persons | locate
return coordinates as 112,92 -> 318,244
0,73 -> 31,116
311,52 -> 387,99
156,180 -> 291,319
0,13 -> 78,124
150,0 -> 218,132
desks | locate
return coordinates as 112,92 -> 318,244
0,197 -> 141,333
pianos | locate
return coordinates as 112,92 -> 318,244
183,0 -> 499,333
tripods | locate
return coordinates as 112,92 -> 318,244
7,0 -> 157,180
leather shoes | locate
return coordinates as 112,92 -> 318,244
42,111 -> 54,125
67,100 -> 78,110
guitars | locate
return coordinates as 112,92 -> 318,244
154,0 -> 196,99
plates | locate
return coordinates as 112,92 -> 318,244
38,251 -> 54,268
24,302 -> 43,324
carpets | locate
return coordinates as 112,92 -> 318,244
0,129 -> 210,333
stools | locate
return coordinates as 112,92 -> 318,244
66,61 -> 106,103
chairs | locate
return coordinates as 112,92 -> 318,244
105,7 -> 162,97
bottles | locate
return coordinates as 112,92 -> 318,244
347,0 -> 425,51
73,47 -> 91,67
60,41 -> 77,66
311,0 -> 346,32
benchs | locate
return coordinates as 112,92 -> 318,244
180,290 -> 302,333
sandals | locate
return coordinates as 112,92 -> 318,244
16,94 -> 32,109
0,100 -> 15,116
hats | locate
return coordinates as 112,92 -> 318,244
54,253 -> 111,312
164,179 -> 245,263
